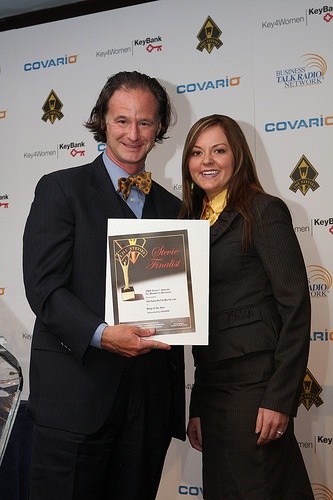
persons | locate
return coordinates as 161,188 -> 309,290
178,114 -> 314,500
23,71 -> 186,500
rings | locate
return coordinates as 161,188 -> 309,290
276,430 -> 284,436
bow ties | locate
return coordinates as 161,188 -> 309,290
117,171 -> 152,201
